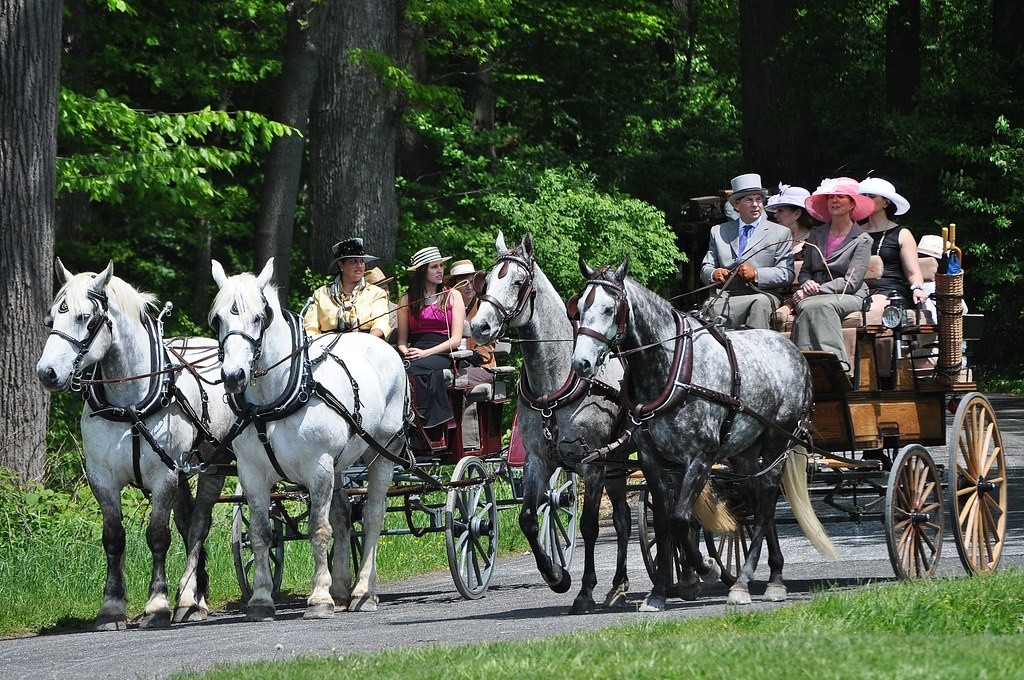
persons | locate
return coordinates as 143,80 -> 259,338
699,173 -> 968,370
303,238 -> 497,448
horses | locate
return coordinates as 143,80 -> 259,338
571,254 -> 813,605
36,253 -> 245,631
473,231 -> 634,616
209,257 -> 410,621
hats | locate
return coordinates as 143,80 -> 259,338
407,246 -> 453,272
916,235 -> 952,259
725,200 -> 768,221
857,177 -> 910,215
763,181 -> 811,213
728,173 -> 768,205
805,176 -> 875,223
329,237 -> 380,276
363,267 -> 395,285
441,260 -> 478,284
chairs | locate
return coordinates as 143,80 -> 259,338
779,257 -> 936,391
448,318 -> 515,400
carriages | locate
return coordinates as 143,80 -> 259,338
469,228 -> 1007,617
34,253 -> 580,634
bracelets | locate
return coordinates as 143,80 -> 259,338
910,283 -> 926,291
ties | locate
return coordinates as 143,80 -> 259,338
738,224 -> 753,264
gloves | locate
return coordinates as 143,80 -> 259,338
736,262 -> 758,283
712,267 -> 733,284
